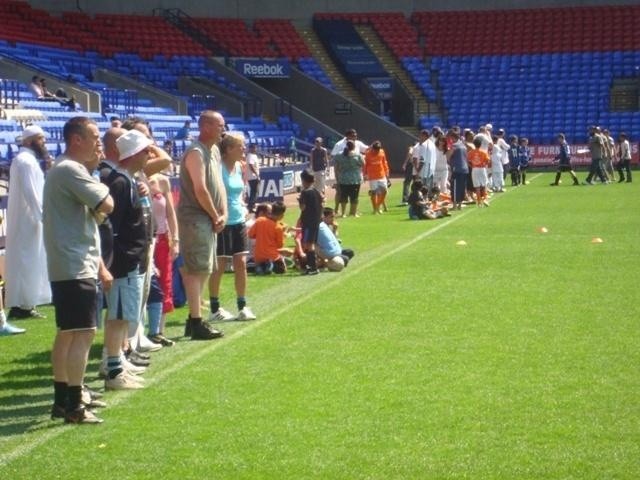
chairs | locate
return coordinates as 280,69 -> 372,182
0,0 -> 640,183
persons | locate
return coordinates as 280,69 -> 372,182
29,76 -> 77,108
550,132 -> 579,186
581,127 -> 632,183
1,110 -> 259,423
311,128 -> 391,218
401,123 -> 532,219
246,168 -> 355,275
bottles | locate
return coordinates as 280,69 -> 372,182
136,182 -> 153,217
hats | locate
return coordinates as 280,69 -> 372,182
116,130 -> 154,161
22,125 -> 43,139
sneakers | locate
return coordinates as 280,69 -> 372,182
210,308 -> 237,321
148,333 -> 175,346
185,319 -> 225,340
100,345 -> 150,389
9,307 -> 47,318
51,386 -> 104,424
301,265 -> 320,275
137,335 -> 162,352
237,307 -> 256,320
0,324 -> 26,336
